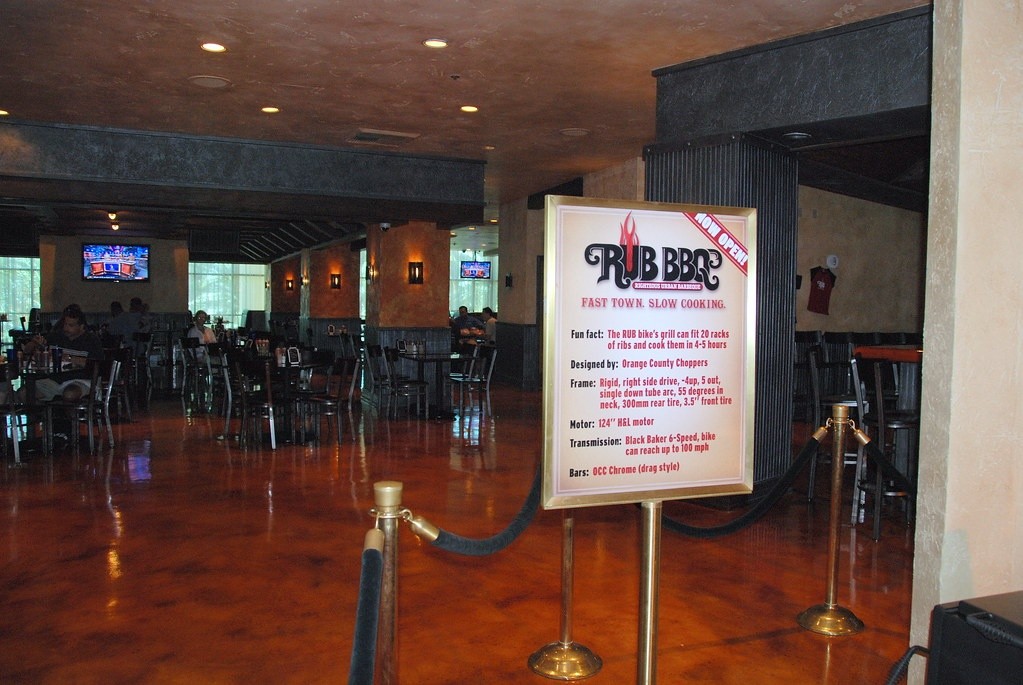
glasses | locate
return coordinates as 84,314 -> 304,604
198,316 -> 206,319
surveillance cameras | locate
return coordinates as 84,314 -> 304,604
379,223 -> 391,232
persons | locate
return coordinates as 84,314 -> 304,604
187,311 -> 220,374
481,307 -> 496,344
18,304 -> 99,442
105,298 -> 150,356
104,252 -> 110,259
454,306 -> 482,328
127,253 -> 134,261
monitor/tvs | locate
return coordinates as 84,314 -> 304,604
81,242 -> 150,283
461,261 -> 491,279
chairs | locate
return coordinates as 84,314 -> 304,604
808,344 -> 919,541
0,311 -> 498,458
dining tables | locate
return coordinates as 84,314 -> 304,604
0,360 -> 79,453
400,353 -> 474,420
273,360 -> 325,440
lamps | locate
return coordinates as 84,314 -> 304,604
286,275 -> 294,291
330,268 -> 341,290
407,255 -> 424,284
107,210 -> 117,220
111,222 -> 120,231
